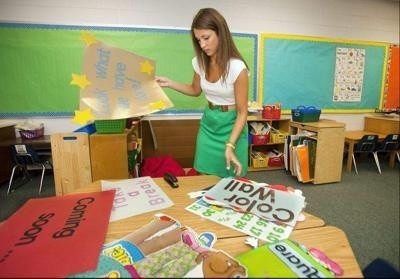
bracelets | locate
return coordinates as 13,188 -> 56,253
226,141 -> 238,151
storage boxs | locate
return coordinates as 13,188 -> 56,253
263,106 -> 280,118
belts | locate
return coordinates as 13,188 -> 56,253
207,101 -> 237,112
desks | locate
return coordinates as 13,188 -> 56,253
365,116 -> 400,134
68,176 -> 364,278
0,123 -> 52,184
345,130 -> 387,173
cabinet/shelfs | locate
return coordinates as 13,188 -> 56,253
52,120 -> 143,195
247,114 -> 345,184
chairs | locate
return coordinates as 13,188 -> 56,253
369,134 -> 400,174
7,144 -> 53,195
352,134 -> 382,176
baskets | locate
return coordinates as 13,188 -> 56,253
261,106 -> 281,119
18,126 -> 44,140
94,118 -> 128,134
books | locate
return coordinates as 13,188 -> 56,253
249,122 -> 317,184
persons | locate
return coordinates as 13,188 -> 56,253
105,231 -> 219,279
156,7 -> 250,179
65,215 -> 190,279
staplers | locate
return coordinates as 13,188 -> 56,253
164,172 -> 179,188
